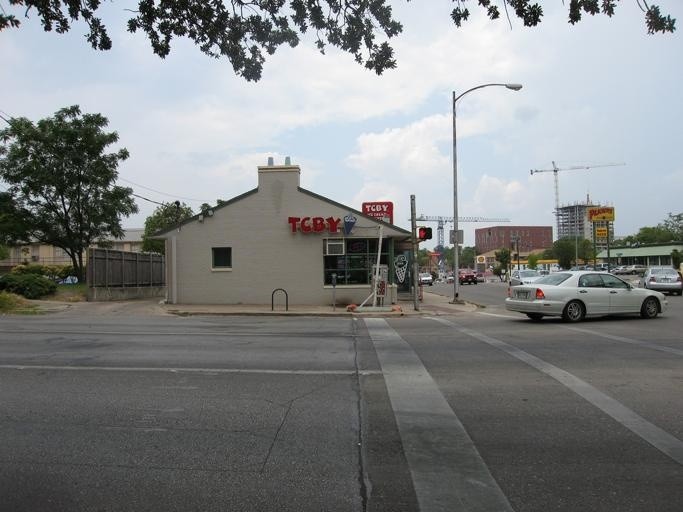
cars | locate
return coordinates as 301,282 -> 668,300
504,263 -> 683,322
418,268 -> 501,286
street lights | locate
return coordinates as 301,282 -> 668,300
451,81 -> 526,305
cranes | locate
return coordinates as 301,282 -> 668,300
407,216 -> 512,273
530,159 -> 628,244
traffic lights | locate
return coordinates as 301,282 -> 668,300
419,227 -> 432,240
